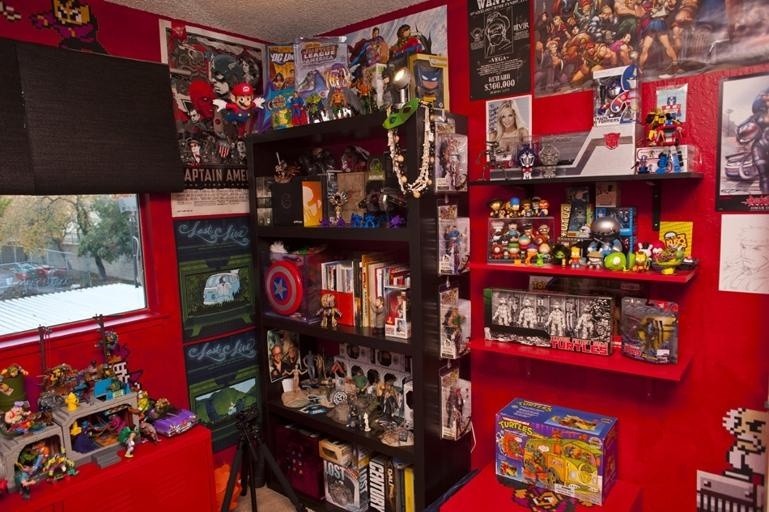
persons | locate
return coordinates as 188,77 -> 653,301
533,0 -> 703,88
481,80 -> 690,365
387,24 -> 422,59
348,27 -> 385,78
1,328 -> 163,504
174,51 -> 465,508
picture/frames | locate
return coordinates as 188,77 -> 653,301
715,70 -> 769,211
485,95 -> 532,161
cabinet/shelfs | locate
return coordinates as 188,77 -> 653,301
466,171 -> 703,382
244,107 -> 479,512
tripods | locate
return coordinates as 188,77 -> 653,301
220,407 -> 308,512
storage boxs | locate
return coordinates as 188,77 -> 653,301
495,397 -> 618,506
